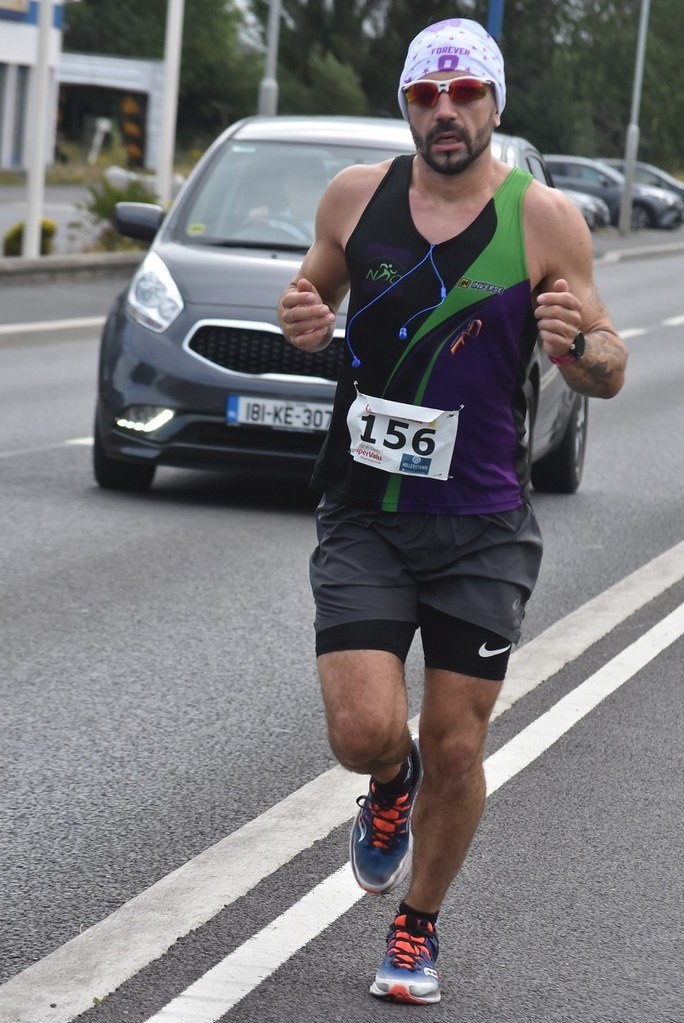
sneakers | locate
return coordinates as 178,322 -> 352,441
369,914 -> 442,1005
349,733 -> 423,895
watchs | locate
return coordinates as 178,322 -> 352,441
549,329 -> 585,368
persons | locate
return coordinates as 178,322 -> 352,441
278,18 -> 627,1004
250,159 -> 328,237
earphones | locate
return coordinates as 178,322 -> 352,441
399,327 -> 408,341
353,356 -> 362,368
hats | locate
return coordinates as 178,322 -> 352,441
398,17 -> 506,122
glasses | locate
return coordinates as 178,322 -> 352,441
401,76 -> 494,107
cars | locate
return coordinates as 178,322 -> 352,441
91,114 -> 590,498
541,153 -> 684,234
553,186 -> 611,232
